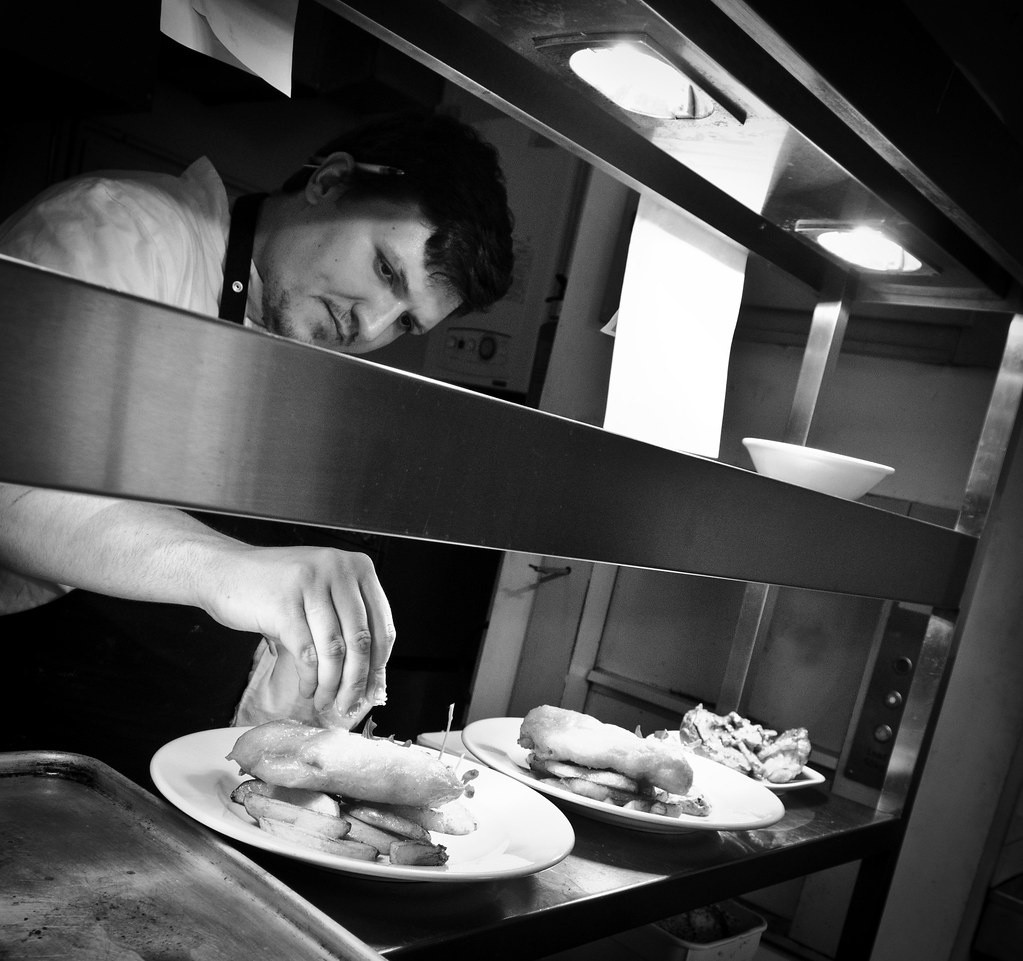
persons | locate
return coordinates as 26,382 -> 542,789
0,107 -> 514,753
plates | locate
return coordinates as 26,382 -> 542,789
150,726 -> 576,881
461,717 -> 785,834
646,730 -> 825,795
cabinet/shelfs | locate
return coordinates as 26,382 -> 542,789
0,0 -> 1023,961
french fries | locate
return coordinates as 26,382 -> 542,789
231,780 -> 478,866
528,750 -> 684,819
633,725 -> 702,754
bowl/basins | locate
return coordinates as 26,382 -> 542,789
742,436 -> 895,500
